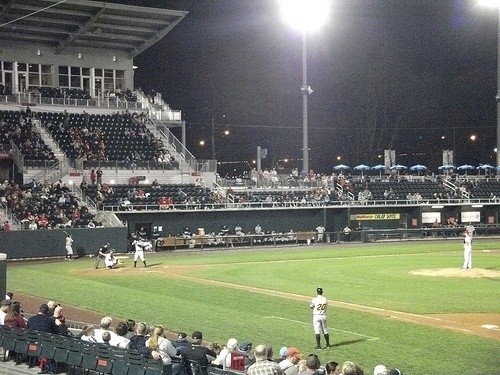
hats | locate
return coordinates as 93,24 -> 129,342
54,307 -> 64,317
237,340 -> 253,353
177,332 -> 188,340
191,331 -> 203,340
101,316 -> 113,324
284,346 -> 303,360
317,287 -> 324,292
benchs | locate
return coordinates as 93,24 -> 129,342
361,223 -> 500,240
157,231 -> 316,249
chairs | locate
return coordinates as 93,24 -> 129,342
229,190 -> 341,208
28,86 -> 96,105
345,179 -> 500,203
0,110 -> 180,171
0,184 -> 227,230
0,324 -> 256,375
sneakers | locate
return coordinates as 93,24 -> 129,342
326,344 -> 331,349
313,345 -> 323,350
64,258 -> 69,261
68,259 -> 74,261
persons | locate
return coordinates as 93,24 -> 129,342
0,88 -> 500,252
132,237 -> 146,267
64,234 -> 74,261
95,242 -> 110,269
310,288 -> 330,350
0,292 -> 402,375
461,232 -> 472,269
105,249 -> 118,269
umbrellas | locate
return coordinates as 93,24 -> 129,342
476,164 -> 494,178
333,164 -> 349,174
371,164 -> 387,178
457,164 -> 475,177
410,164 -> 428,176
390,164 -> 407,178
438,165 -> 456,176
354,164 -> 370,176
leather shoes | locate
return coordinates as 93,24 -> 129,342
95,266 -> 100,269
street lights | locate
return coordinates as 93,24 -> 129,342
294,0 -> 317,174
